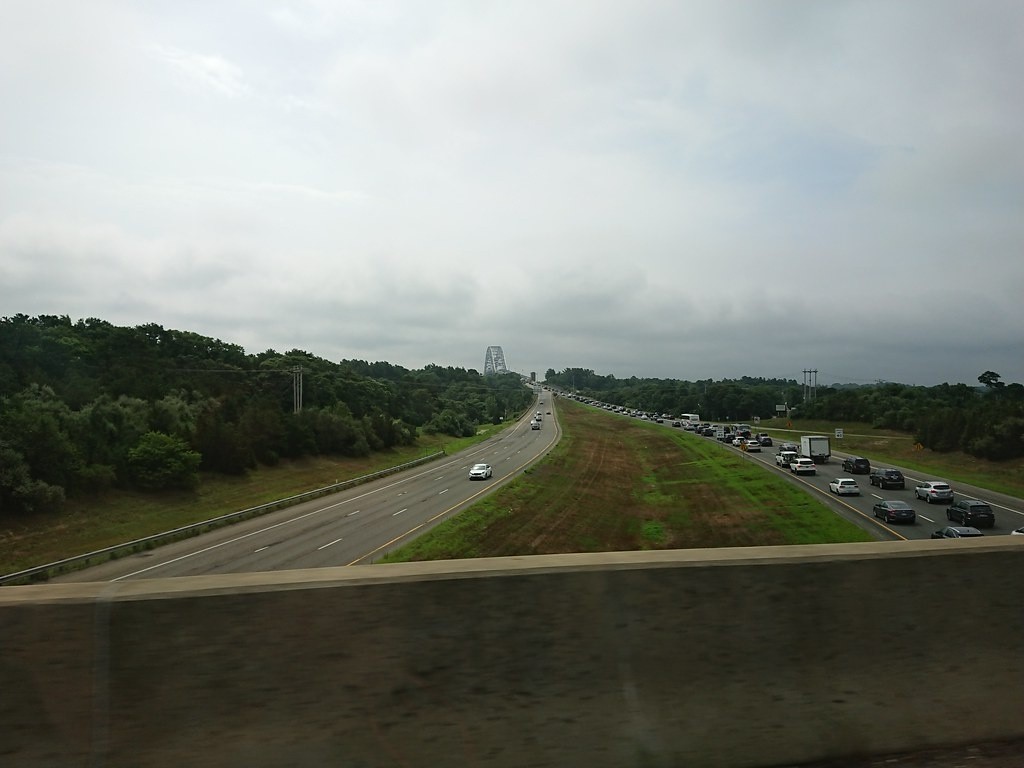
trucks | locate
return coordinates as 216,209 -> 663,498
800,436 -> 832,464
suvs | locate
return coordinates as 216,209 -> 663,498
946,499 -> 995,528
775,451 -> 800,468
869,469 -> 905,490
841,456 -> 871,474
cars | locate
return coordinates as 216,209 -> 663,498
530,381 -> 773,452
540,401 -> 543,404
931,525 -> 984,539
468,463 -> 493,480
915,481 -> 954,503
779,443 -> 798,451
789,458 -> 816,476
873,501 -> 916,524
829,478 -> 861,496
1011,527 -> 1024,536
546,411 -> 551,415
